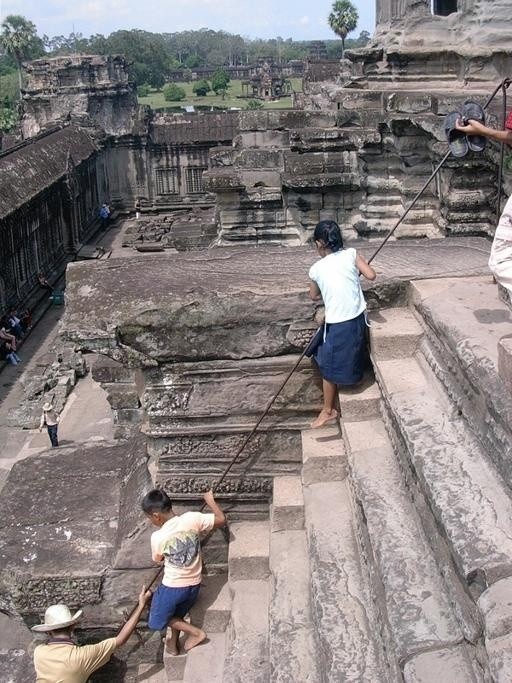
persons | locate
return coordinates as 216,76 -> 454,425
37,271 -> 54,296
31,586 -> 153,683
141,487 -> 229,656
38,403 -> 60,447
100,202 -> 112,231
307,218 -> 377,430
454,91 -> 512,311
0,306 -> 32,366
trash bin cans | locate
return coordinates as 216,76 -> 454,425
52,290 -> 63,305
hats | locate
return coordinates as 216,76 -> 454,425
31,604 -> 83,631
43,403 -> 52,410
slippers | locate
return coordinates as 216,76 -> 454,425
444,99 -> 487,158
